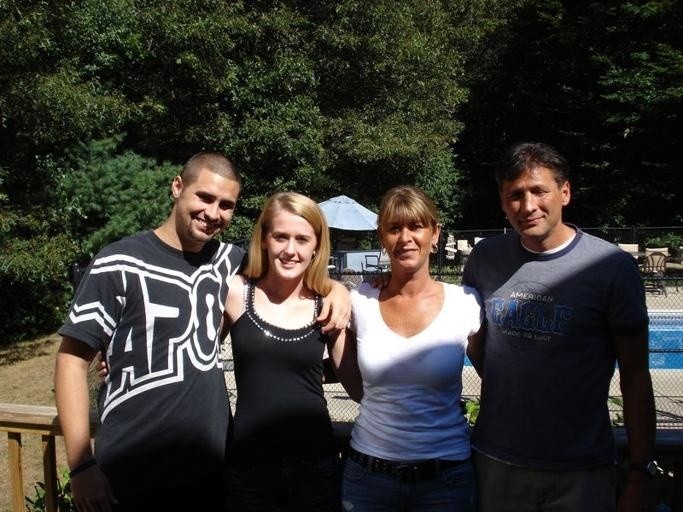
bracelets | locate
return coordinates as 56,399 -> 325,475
67,456 -> 97,479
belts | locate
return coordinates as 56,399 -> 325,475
348,443 -> 466,481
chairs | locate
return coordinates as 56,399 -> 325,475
617,243 -> 683,297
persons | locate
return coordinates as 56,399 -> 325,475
50,150 -> 353,510
455,140 -> 659,512
325,184 -> 485,509
218,191 -> 354,509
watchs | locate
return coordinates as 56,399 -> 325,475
623,455 -> 659,479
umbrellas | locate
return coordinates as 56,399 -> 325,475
310,193 -> 380,259
